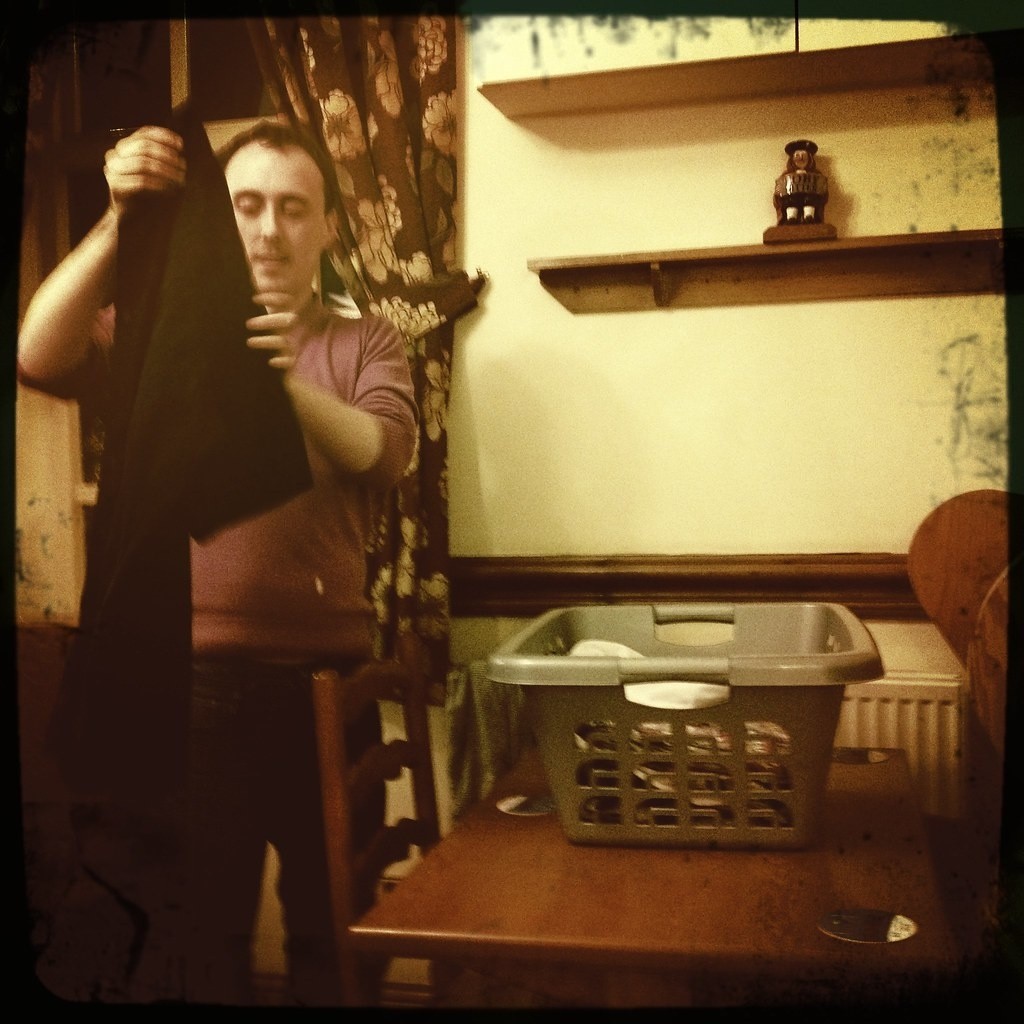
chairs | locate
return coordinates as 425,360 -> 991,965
311,626 -> 446,1011
832,670 -> 969,821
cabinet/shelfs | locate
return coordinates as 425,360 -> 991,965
474,25 -> 1019,312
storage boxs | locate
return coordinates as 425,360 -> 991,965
487,603 -> 883,851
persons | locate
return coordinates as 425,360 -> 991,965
773,139 -> 829,226
18,121 -> 420,1009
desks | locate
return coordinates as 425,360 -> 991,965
342,736 -> 959,1023
